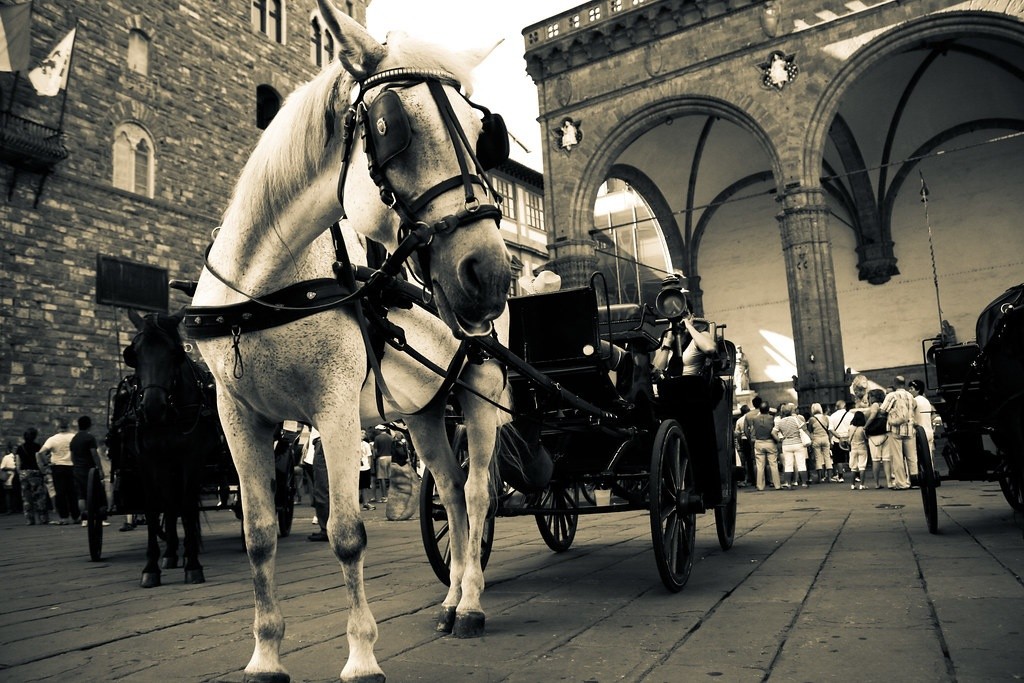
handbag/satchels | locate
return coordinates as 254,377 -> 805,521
796,418 -> 811,446
867,413 -> 888,435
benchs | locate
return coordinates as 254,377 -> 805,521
934,344 -> 985,393
507,281 -> 735,509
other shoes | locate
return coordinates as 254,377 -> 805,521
119,523 -> 134,531
102,521 -> 110,525
81,520 -> 87,526
742,474 -> 889,490
308,532 -> 328,542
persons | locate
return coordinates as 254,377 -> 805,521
880,376 -> 920,491
863,388 -> 895,488
808,402 -> 835,483
39,418 -> 83,526
828,400 -> 860,482
752,401 -> 784,491
907,379 -> 936,490
0,302 -> 956,537
70,417 -> 111,527
15,427 -> 53,525
848,410 -> 870,490
650,300 -> 718,404
771,404 -> 811,488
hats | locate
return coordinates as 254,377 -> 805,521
375,424 -> 386,429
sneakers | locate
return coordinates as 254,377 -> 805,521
363,503 -> 376,510
377,497 -> 387,502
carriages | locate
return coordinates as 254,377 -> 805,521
85,306 -> 298,588
170,0 -> 751,682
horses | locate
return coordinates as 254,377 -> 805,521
122,308 -> 214,586
186,0 -> 513,683
